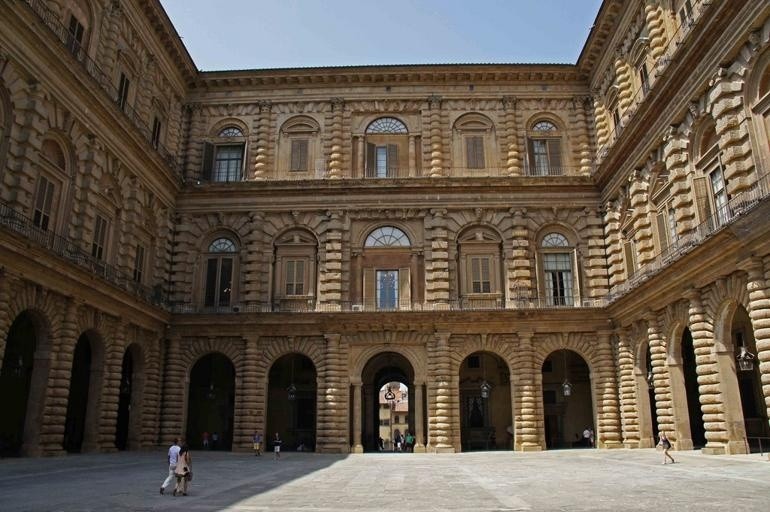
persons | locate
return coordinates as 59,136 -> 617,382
203,430 -> 209,450
173,443 -> 192,496
584,427 -> 590,447
259,433 -> 265,453
659,429 -> 674,464
380,430 -> 414,452
158,436 -> 183,495
212,432 -> 219,448
253,430 -> 261,456
589,427 -> 595,448
273,432 -> 283,460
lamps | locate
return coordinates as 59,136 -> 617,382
478,349 -> 493,400
285,351 -> 299,401
732,296 -> 759,375
559,347 -> 577,397
